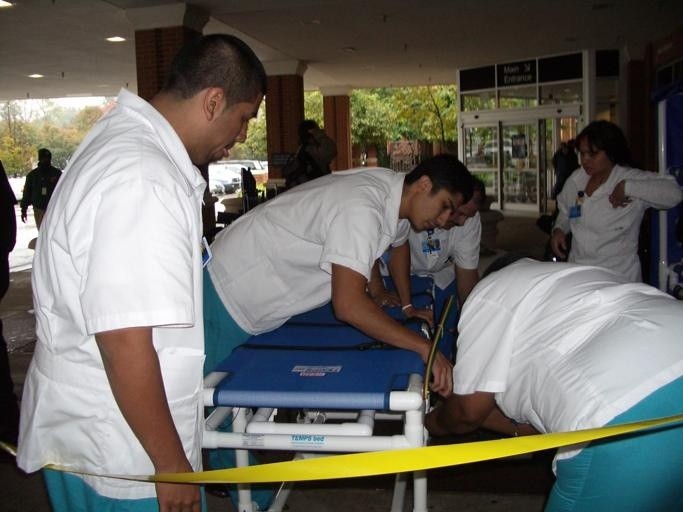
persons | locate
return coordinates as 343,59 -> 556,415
281,119 -> 338,192
0,159 -> 20,464
19,148 -> 63,232
16,30 -> 269,512
366,174 -> 486,309
200,152 -> 476,511
424,250 -> 683,512
549,119 -> 683,282
544,139 -> 581,262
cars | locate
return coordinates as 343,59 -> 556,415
206,158 -> 270,199
485,139 -> 513,161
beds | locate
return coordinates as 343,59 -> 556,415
203,276 -> 455,512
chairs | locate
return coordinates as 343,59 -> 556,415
241,168 -> 264,215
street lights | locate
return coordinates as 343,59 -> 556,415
468,125 -> 477,158
